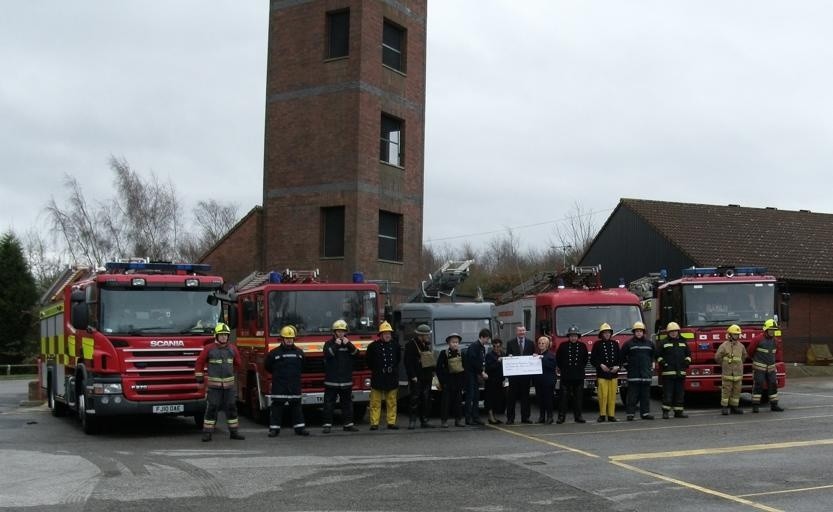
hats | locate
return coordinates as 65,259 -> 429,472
445,333 -> 462,342
414,324 -> 432,335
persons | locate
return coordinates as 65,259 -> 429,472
321,320 -> 360,433
748,319 -> 784,413
655,322 -> 692,419
264,326 -> 310,437
366,320 -> 402,431
195,323 -> 245,442
715,324 -> 749,415
405,324 -> 555,430
590,323 -> 623,422
555,325 -> 588,424
621,321 -> 656,421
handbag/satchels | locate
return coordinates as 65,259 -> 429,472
411,339 -> 436,368
446,349 -> 465,373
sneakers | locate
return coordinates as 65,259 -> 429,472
369,425 -> 378,430
229,433 -> 245,440
269,430 -> 279,436
343,427 -> 359,431
201,432 -> 212,441
597,415 -> 619,422
627,415 -> 634,421
642,415 -> 656,420
387,425 -> 400,429
295,430 -> 309,435
323,427 -> 331,433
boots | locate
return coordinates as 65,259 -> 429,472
772,401 -> 784,412
674,411 -> 688,418
662,410 -> 670,419
408,412 -> 586,428
731,406 -> 743,415
753,404 -> 760,413
722,406 -> 729,415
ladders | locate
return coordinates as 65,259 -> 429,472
628,272 -> 662,295
227,268 -> 275,296
495,264 -> 555,305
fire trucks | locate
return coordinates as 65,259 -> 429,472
382,257 -> 503,413
497,261 -> 645,406
624,265 -> 789,399
39,261 -> 225,433
223,268 -> 381,424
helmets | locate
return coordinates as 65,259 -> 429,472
632,322 -> 645,332
598,323 -> 613,333
727,324 -> 742,334
215,323 -> 231,335
667,321 -> 681,332
280,327 -> 295,338
332,319 -> 349,332
378,321 -> 394,333
763,319 -> 779,330
